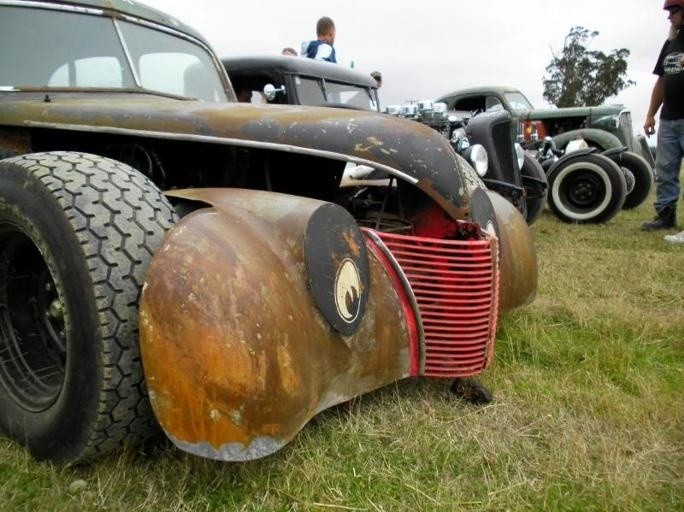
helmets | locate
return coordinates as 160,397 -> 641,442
663,0 -> 684,11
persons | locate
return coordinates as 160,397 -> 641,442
642,0 -> 684,231
282,16 -> 336,64
345,71 -> 383,109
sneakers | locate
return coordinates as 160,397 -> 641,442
663,230 -> 684,244
641,207 -> 677,230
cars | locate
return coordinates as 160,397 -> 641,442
183,53 -> 549,227
433,85 -> 657,226
0,0 -> 540,471
